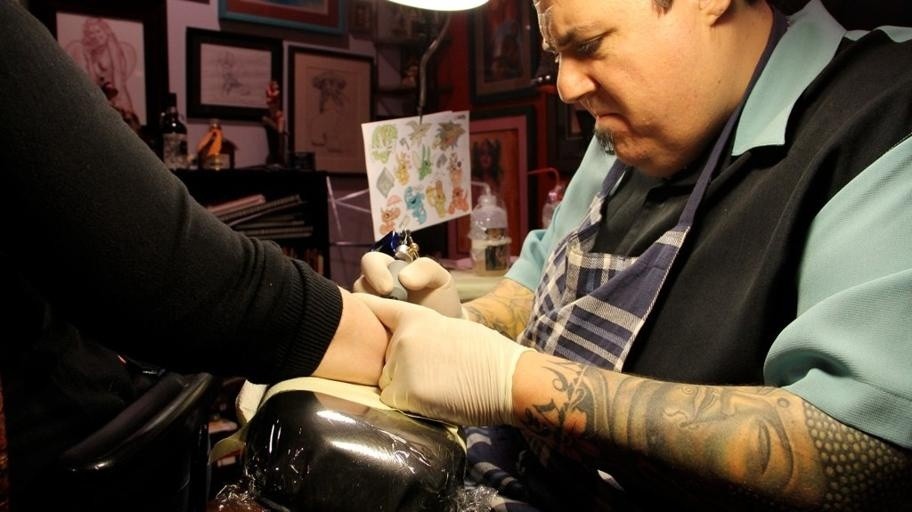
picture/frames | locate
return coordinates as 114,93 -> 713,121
468,1 -> 541,107
282,39 -> 378,179
185,26 -> 283,124
26,0 -> 169,163
447,103 -> 538,271
544,91 -> 596,180
218,0 -> 345,37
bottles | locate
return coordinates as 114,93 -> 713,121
542,192 -> 564,229
208,118 -> 224,170
470,192 -> 512,277
158,90 -> 190,171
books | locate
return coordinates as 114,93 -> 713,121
203,190 -> 325,278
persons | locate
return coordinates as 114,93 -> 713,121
0,0 -> 393,510
80,15 -> 140,133
350,0 -> 912,508
470,136 -> 508,206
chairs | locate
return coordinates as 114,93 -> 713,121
0,356 -> 213,512
